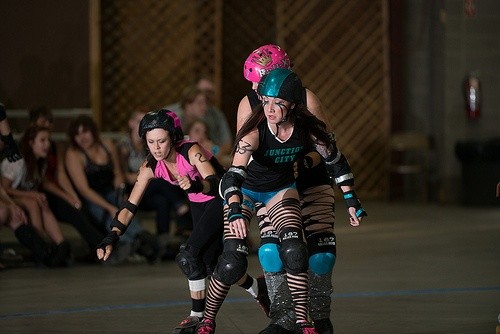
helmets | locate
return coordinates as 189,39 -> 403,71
138,109 -> 183,142
257,67 -> 303,106
243,45 -> 291,81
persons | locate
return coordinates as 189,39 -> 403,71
0,45 -> 335,334
195,68 -> 368,334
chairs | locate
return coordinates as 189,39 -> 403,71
383,131 -> 430,202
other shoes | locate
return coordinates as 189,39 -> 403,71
296,321 -> 317,334
195,318 -> 218,334
172,314 -> 206,334
254,276 -> 271,318
0,231 -> 188,272
313,317 -> 334,334
256,323 -> 304,334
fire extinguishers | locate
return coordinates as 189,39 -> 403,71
465,74 -> 480,121
